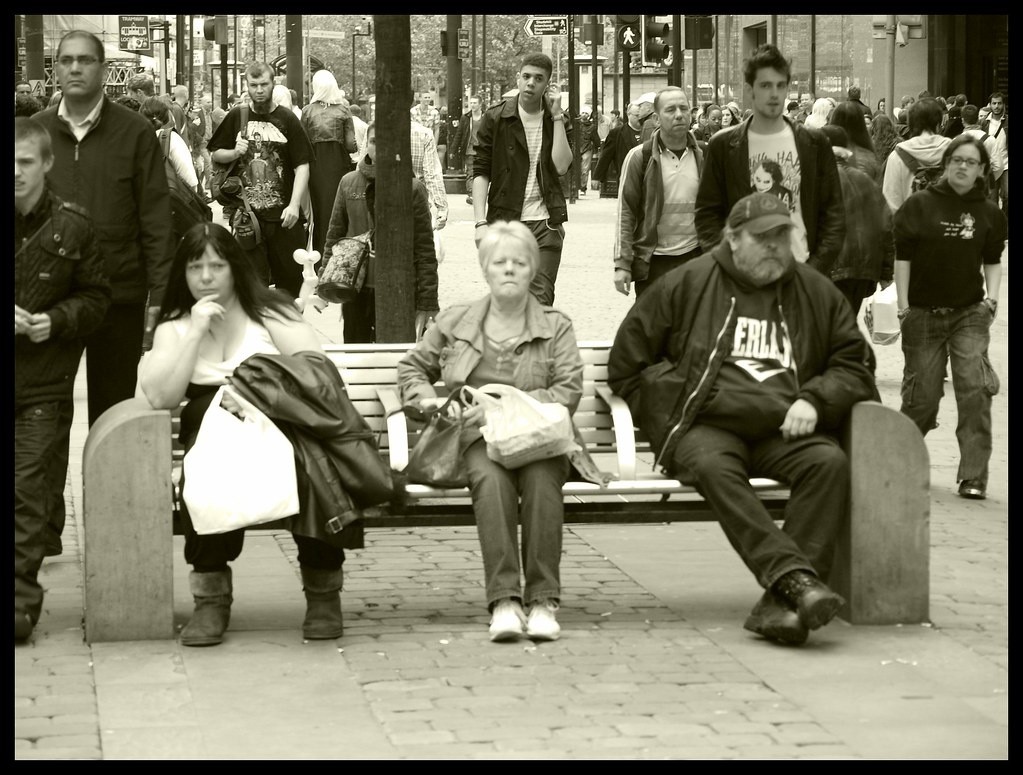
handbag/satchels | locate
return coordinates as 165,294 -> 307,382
182,384 -> 300,536
460,384 -> 584,470
864,282 -> 901,346
376,386 -> 483,489
318,230 -> 371,304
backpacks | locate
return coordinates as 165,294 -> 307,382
210,102 -> 249,206
895,145 -> 944,194
157,127 -> 213,237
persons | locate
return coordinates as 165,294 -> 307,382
689,101 -> 753,143
311,124 -> 440,345
470,55 -> 575,306
397,222 -> 606,642
36,28 -> 169,554
359,87 -> 491,230
894,134 -> 1004,499
608,195 -> 876,642
15,117 -> 100,637
116,63 -> 373,313
611,87 -> 707,295
561,96 -> 657,200
694,47 -> 845,278
787,86 -> 1008,382
15,82 -> 61,117
139,221 -> 345,643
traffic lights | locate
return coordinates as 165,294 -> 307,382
642,13 -> 670,66
614,14 -> 641,52
204,14 -> 228,45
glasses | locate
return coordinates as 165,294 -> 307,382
947,155 -> 982,169
56,56 -> 104,66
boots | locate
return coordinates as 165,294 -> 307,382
301,566 -> 343,640
180,566 -> 234,646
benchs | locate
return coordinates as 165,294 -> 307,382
81,344 -> 931,640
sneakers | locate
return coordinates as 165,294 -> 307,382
526,599 -> 561,642
774,569 -> 847,631
743,588 -> 808,646
488,596 -> 531,641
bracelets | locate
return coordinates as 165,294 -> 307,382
475,220 -> 488,227
898,308 -> 909,319
987,297 -> 998,307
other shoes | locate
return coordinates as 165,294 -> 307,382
15,608 -> 34,643
43,527 -> 63,556
958,479 -> 986,500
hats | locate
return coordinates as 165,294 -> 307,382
634,92 -> 657,105
729,193 -> 793,237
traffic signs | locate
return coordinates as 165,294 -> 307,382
523,16 -> 569,37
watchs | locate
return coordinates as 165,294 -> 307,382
552,113 -> 564,121
